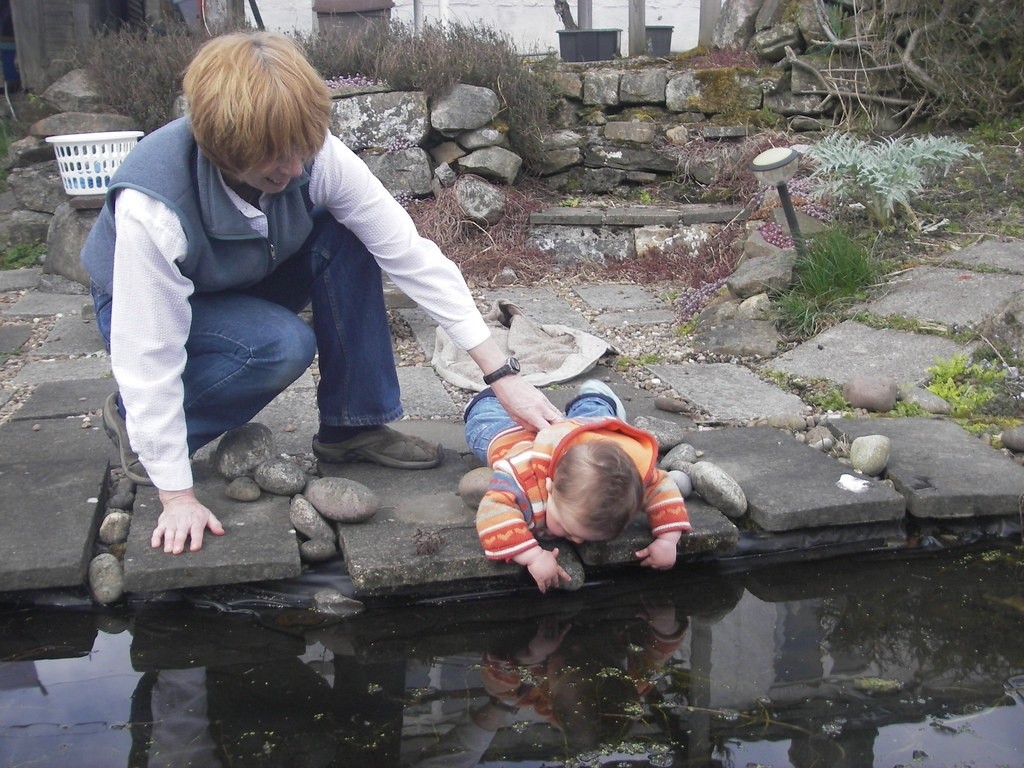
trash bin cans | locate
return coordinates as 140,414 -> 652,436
556,28 -> 623,62
643,25 -> 674,55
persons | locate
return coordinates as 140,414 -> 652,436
127,651 -> 510,768
462,379 -> 691,594
77,31 -> 564,555
481,597 -> 690,743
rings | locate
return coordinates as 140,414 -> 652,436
550,413 -> 558,422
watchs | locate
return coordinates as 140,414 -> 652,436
483,356 -> 521,385
489,696 -> 520,715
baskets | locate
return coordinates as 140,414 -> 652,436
46,131 -> 144,195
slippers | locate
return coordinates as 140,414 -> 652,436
312,424 -> 442,469
101,392 -> 157,485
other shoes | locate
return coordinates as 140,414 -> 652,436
581,379 -> 627,424
